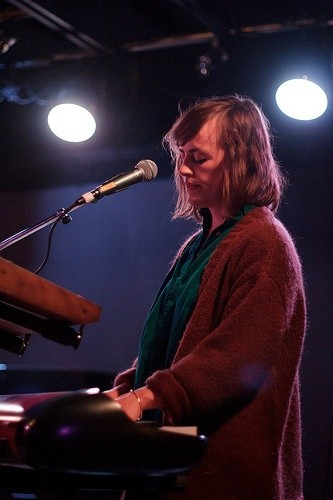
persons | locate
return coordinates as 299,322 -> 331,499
101,95 -> 306,500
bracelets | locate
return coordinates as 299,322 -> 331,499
130,389 -> 142,421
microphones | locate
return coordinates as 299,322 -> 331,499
75,161 -> 157,208
